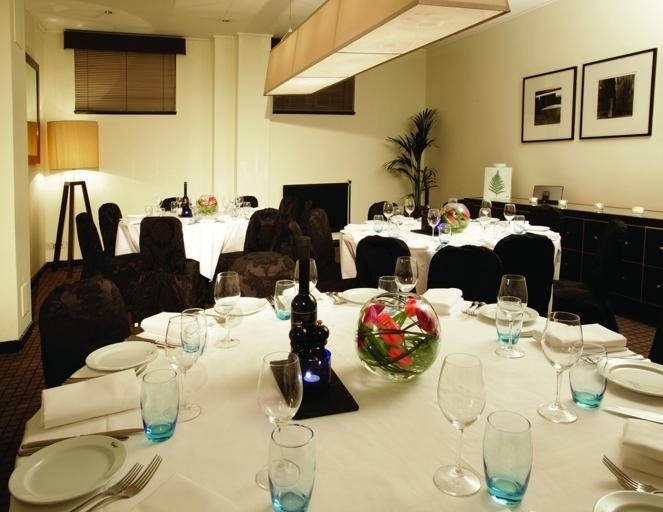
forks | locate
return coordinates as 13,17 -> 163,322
265,295 -> 276,311
333,292 -> 347,303
462,302 -> 478,313
326,292 -> 341,304
81,455 -> 162,512
603,456 -> 663,494
70,462 -> 144,512
469,301 -> 486,315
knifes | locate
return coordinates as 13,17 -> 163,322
603,403 -> 663,424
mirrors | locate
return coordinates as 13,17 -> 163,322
26,52 -> 40,165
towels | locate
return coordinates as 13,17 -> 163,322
423,287 -> 463,315
533,322 -> 627,354
621,420 -> 663,479
141,311 -> 199,334
42,368 -> 140,429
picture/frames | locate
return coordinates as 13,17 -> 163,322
579,47 -> 657,140
521,66 -> 577,143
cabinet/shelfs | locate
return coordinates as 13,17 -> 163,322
458,198 -> 663,324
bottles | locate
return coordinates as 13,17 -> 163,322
182,182 -> 188,211
421,186 -> 431,232
290,236 -> 316,328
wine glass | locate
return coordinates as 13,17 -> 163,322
537,312 -> 583,423
164,315 -> 201,422
495,274 -> 528,358
214,272 -> 241,347
384,202 -> 393,223
433,353 -> 487,497
478,208 -> 492,232
392,207 -> 403,232
482,200 -> 492,208
405,199 -> 415,218
428,208 -> 441,239
395,256 -> 419,292
256,352 -> 304,489
295,258 -> 318,295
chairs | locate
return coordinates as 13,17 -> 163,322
38,275 -> 132,385
493,233 -> 555,318
76,212 -> 142,296
368,201 -> 398,220
427,245 -> 504,304
140,216 -> 199,314
356,235 -> 413,291
98,203 -> 122,255
219,208 -> 297,294
553,232 -> 618,333
308,207 -> 359,292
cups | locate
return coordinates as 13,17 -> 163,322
378,276 -> 399,294
374,215 -> 383,233
569,343 -> 607,408
269,425 -> 316,512
504,204 -> 516,226
513,215 -> 526,234
146,200 -> 200,223
216,193 -> 251,222
274,280 -> 297,320
438,223 -> 451,246
140,369 -> 179,441
496,297 -> 525,344
182,308 -> 206,352
484,411 -> 533,507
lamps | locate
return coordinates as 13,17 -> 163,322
44,120 -> 100,279
264,1 -> 510,96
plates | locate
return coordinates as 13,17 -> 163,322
342,288 -> 378,303
599,359 -> 663,396
9,435 -> 128,503
479,303 -> 539,322
214,297 -> 265,315
595,491 -> 663,512
86,341 -> 158,371
